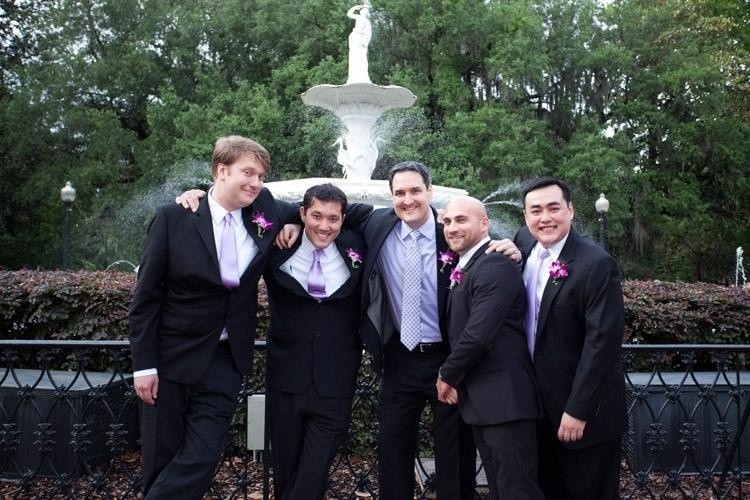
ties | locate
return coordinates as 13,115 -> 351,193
399,230 -> 423,352
450,262 -> 462,290
524,246 -> 551,364
307,247 -> 330,303
218,212 -> 240,291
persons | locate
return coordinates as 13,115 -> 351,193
123,132 -> 389,500
432,193 -> 547,498
274,159 -> 527,500
507,176 -> 630,498
175,180 -> 445,500
344,3 -> 372,83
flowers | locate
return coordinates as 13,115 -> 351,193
447,267 -> 464,292
547,259 -> 570,286
252,211 -> 273,241
343,247 -> 363,270
436,249 -> 456,273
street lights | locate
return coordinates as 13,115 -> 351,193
59,178 -> 79,270
594,191 -> 609,250
